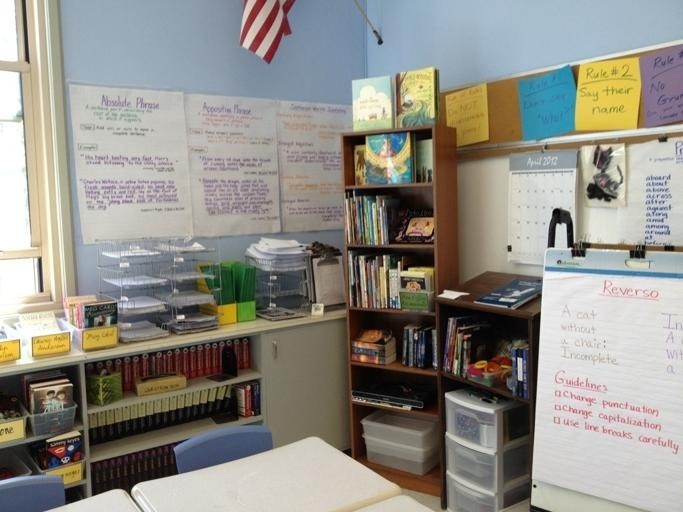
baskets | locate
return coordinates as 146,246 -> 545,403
0,449 -> 32,482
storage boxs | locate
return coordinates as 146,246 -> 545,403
16,402 -> 78,437
446,389 -> 530,449
362,434 -> 438,475
447,471 -> 531,512
446,432 -> 533,492
58,317 -> 118,351
0,450 -> 32,477
0,320 -> 21,363
360,410 -> 439,462
14,319 -> 72,358
0,400 -> 26,445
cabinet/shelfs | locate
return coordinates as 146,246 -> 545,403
434,271 -> 542,468
340,124 -> 445,511
0,354 -> 89,497
263,319 -> 351,451
83,321 -> 264,463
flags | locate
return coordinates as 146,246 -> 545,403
237,0 -> 298,65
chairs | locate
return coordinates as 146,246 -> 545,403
0,475 -> 67,512
174,424 -> 273,474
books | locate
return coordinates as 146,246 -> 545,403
20,369 -> 81,467
442,313 -> 531,400
84,337 -> 262,494
351,329 -> 397,366
401,323 -> 437,371
396,66 -> 439,128
348,249 -> 436,314
347,131 -> 433,184
473,278 -> 542,311
351,75 -> 397,131
343,188 -> 435,245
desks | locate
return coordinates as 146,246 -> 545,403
349,495 -> 438,512
131,435 -> 401,512
44,487 -> 140,512
